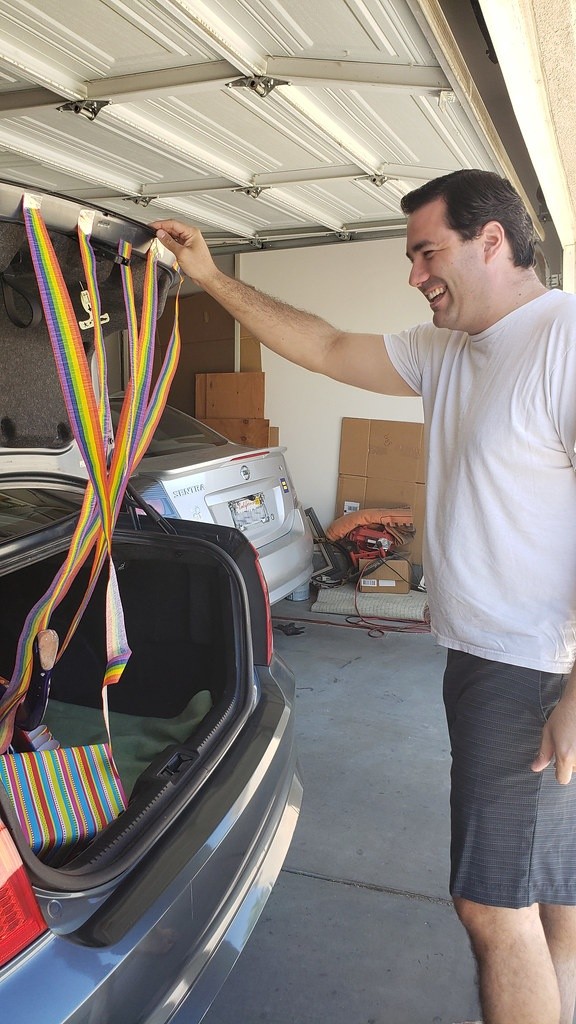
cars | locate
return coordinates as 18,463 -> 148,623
0,175 -> 305,1024
106,396 -> 314,607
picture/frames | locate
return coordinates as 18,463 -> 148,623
303,507 -> 342,583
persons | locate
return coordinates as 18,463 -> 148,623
147,169 -> 575,1024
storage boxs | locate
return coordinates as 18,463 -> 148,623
359,552 -> 412,594
